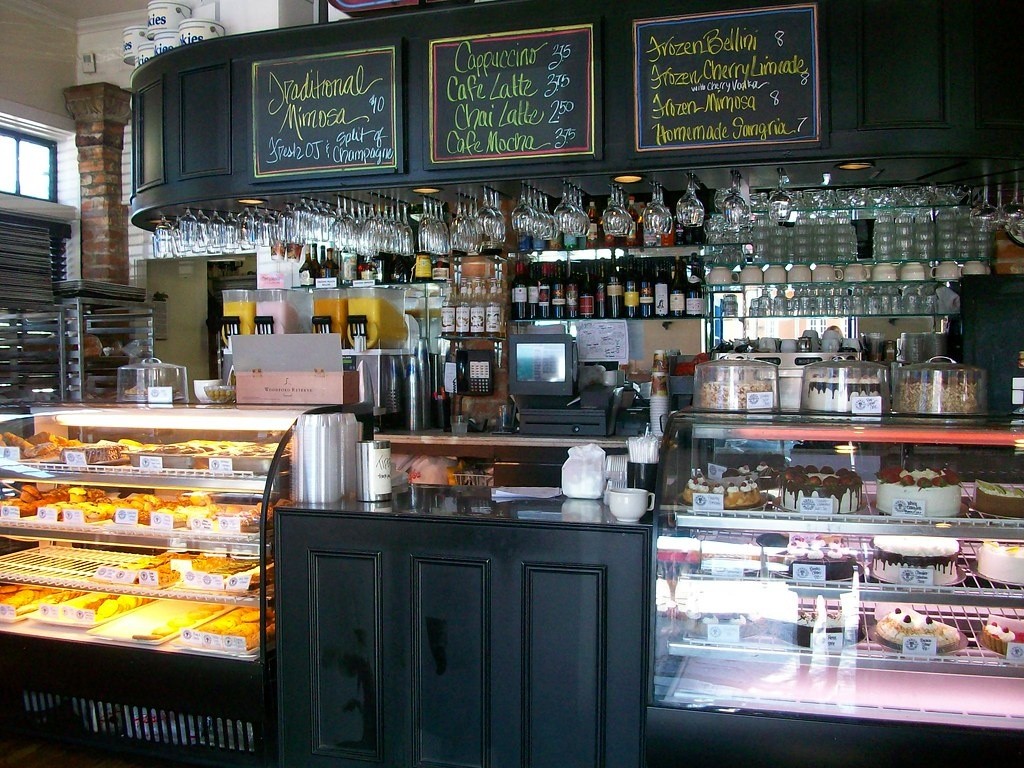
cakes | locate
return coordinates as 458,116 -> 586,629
972,479 -> 1024,518
656,532 -> 1024,660
701,381 -> 774,408
892,375 -> 980,414
681,461 -> 863,513
873,466 -> 962,517
808,371 -> 881,412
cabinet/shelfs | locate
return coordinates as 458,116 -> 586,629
0,299 -> 159,407
0,442 -> 290,661
435,203 -> 995,367
655,480 -> 1024,733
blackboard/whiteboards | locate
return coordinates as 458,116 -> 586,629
627,0 -> 830,160
421,16 -> 604,171
247,35 -> 410,185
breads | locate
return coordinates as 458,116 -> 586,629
67,334 -> 122,400
0,430 -> 292,652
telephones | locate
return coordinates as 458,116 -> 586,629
456,349 -> 493,396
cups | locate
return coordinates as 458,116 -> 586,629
291,412 -> 359,505
625,461 -> 659,504
195,379 -> 225,405
650,349 -> 670,437
354,440 -> 392,502
867,328 -> 948,365
450,413 -> 468,434
707,260 -> 993,285
733,325 -> 862,352
497,404 -> 512,429
608,487 -> 654,523
404,339 -> 440,431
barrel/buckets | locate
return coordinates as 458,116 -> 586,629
121,2 -> 225,76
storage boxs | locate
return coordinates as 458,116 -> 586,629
312,288 -> 347,340
254,289 -> 313,334
231,333 -> 359,404
346,289 -> 409,339
222,289 -> 257,335
120,440 -> 290,474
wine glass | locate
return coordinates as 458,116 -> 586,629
152,169 -> 1024,262
720,283 -> 940,315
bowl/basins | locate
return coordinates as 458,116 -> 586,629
203,384 -> 237,405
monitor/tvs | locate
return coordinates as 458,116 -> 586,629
508,333 -> 574,396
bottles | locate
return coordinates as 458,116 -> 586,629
516,196 -> 677,253
298,242 -> 708,341
